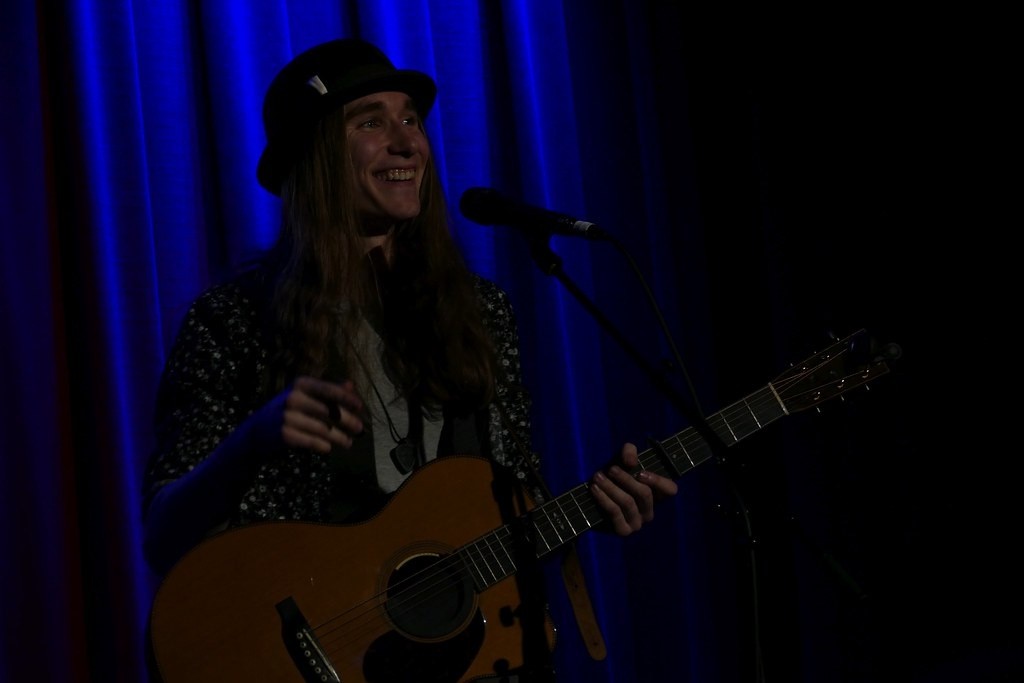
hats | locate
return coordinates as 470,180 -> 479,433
255,36 -> 439,197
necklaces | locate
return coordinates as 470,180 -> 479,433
333,315 -> 424,475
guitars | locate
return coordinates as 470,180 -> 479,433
149,322 -> 900,682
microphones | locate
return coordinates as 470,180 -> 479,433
458,184 -> 601,239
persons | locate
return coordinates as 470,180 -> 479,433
144,37 -> 679,683
